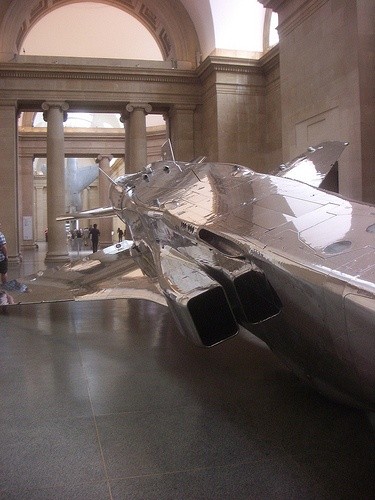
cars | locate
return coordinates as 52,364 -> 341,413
65,223 -> 71,233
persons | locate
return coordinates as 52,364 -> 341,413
117,228 -> 123,243
0,279 -> 32,293
0,232 -> 8,283
90,224 -> 100,253
71,226 -> 92,246
